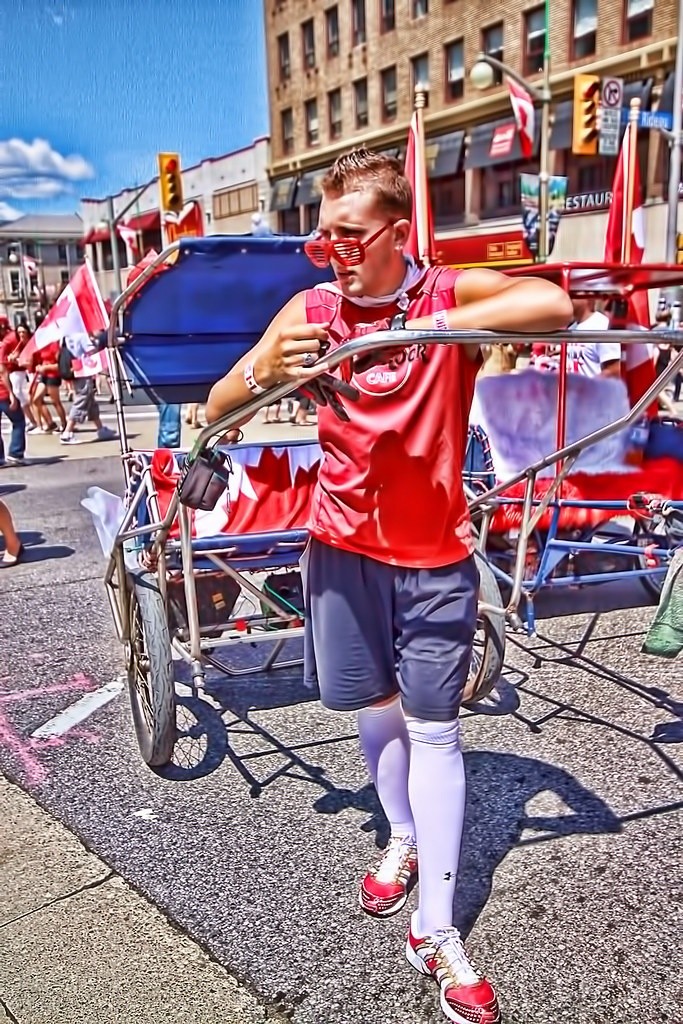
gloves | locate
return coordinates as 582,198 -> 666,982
330,318 -> 411,385
293,339 -> 361,422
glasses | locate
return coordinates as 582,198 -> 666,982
305,222 -> 393,268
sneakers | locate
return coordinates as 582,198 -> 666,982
405,909 -> 501,1024
359,836 -> 418,915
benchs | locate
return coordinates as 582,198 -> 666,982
467,373 -> 682,539
129,421 -> 494,567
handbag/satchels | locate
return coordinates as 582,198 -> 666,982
57,338 -> 75,380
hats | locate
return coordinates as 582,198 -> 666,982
0,316 -> 11,330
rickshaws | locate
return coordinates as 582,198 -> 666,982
79,214 -> 682,767
463,262 -> 683,637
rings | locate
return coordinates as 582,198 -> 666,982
302,352 -> 311,363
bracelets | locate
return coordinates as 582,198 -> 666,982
245,359 -> 267,395
432,309 -> 447,330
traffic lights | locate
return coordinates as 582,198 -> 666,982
158,153 -> 182,211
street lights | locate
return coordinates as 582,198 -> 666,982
470,47 -> 552,264
6,239 -> 32,332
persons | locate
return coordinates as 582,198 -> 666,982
61,360 -> 119,444
206,146 -> 575,1023
0,499 -> 25,568
567,287 -> 622,384
0,316 -> 66,466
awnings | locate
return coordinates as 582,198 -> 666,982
81,210 -> 161,243
267,71 -> 675,209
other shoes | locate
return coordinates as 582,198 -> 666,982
98,427 -> 118,440
60,432 -> 81,444
1,543 -> 24,567
7,452 -> 27,464
23,423 -> 66,435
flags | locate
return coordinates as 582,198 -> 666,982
604,121 -> 653,329
402,112 -> 436,260
506,75 -> 534,157
20,263 -> 108,362
